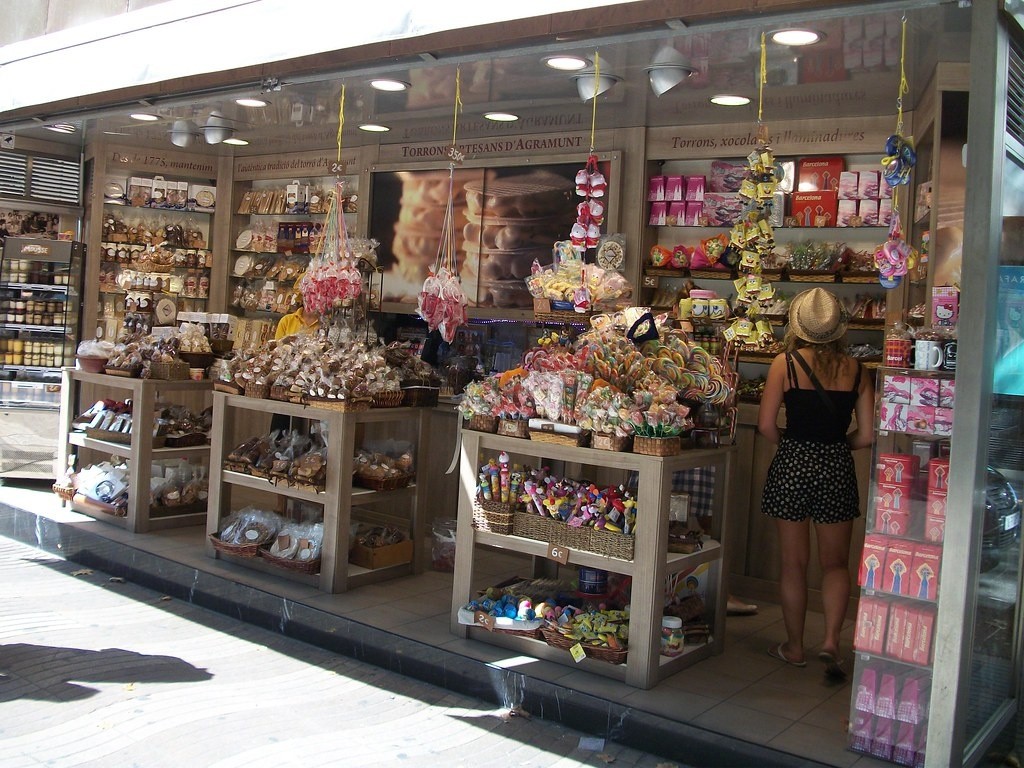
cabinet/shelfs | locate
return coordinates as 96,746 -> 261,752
81,140 -> 230,342
635,116 -> 913,412
203,390 -> 433,595
56,366 -> 233,533
450,426 -> 741,690
846,365 -> 958,768
226,146 -> 371,353
0,236 -> 88,410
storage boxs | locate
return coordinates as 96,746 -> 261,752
52,151 -> 957,768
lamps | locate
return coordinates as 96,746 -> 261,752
570,75 -> 623,103
644,66 -> 693,98
167,131 -> 203,148
200,127 -> 237,145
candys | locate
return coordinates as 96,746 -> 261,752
527,240 -> 631,311
457,312 -> 732,439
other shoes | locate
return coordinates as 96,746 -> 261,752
726,600 -> 759,616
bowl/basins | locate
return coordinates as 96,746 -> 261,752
74,354 -> 108,372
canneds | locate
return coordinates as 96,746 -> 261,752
0,258 -> 77,368
101,241 -> 205,334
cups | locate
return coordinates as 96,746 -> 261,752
915,341 -> 943,371
886,340 -> 911,367
190,368 -> 204,381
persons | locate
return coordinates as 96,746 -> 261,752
270,302 -> 329,519
759,287 -> 874,683
628,464 -> 757,614
0,210 -> 59,247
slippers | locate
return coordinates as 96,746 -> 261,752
817,650 -> 846,682
767,643 -> 807,667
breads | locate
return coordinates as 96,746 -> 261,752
77,322 -> 214,514
226,332 -> 438,487
229,190 -> 358,313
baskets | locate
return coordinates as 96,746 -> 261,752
371,390 -> 404,409
213,374 -> 370,415
456,604 -> 541,642
222,457 -> 274,480
208,336 -> 234,352
353,471 -> 417,491
209,531 -> 258,560
165,432 -> 207,448
52,483 -> 78,501
539,626 -> 628,665
101,363 -> 142,378
532,303 -> 602,328
470,412 -> 683,455
469,495 -> 699,562
85,425 -> 166,454
643,264 -> 888,365
131,258 -> 175,273
148,359 -> 191,382
256,538 -> 321,576
402,377 -> 442,407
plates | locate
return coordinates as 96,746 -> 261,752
235,255 -> 250,276
237,230 -> 252,248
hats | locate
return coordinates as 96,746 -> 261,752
788,287 -> 849,344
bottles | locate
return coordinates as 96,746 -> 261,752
0,260 -> 77,367
660,615 -> 683,656
123,271 -> 171,334
